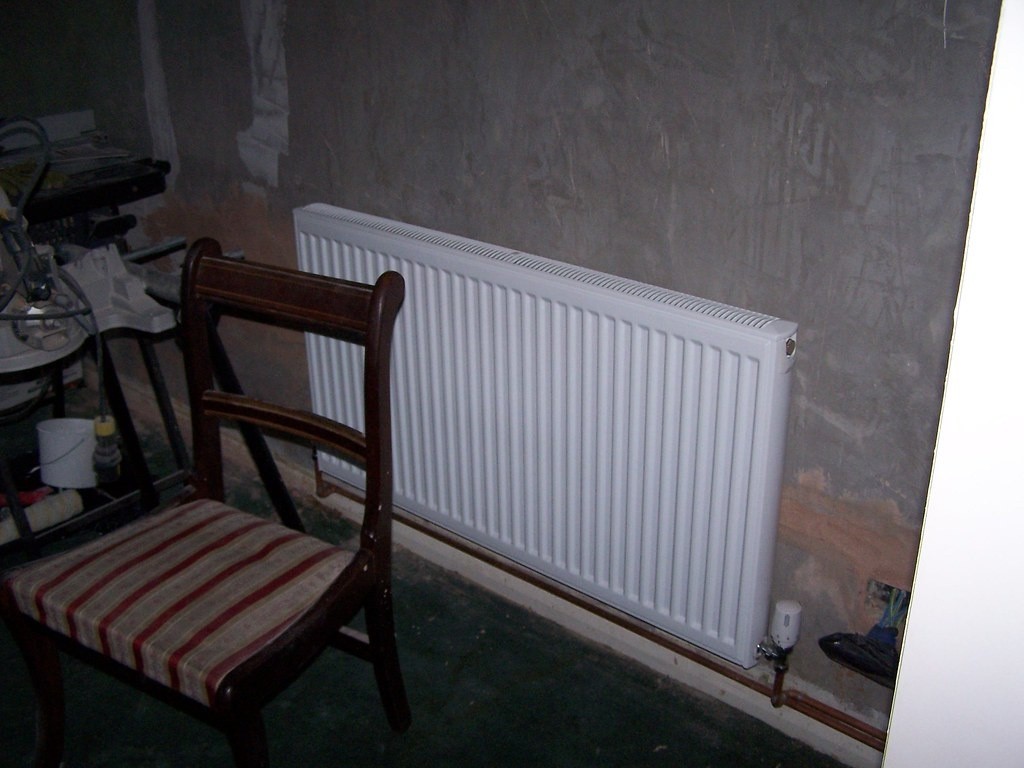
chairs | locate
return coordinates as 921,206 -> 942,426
0,235 -> 412,768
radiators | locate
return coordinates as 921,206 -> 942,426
294,203 -> 799,670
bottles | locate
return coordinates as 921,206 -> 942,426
92,415 -> 123,493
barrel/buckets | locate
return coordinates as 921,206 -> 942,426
37,418 -> 96,488
